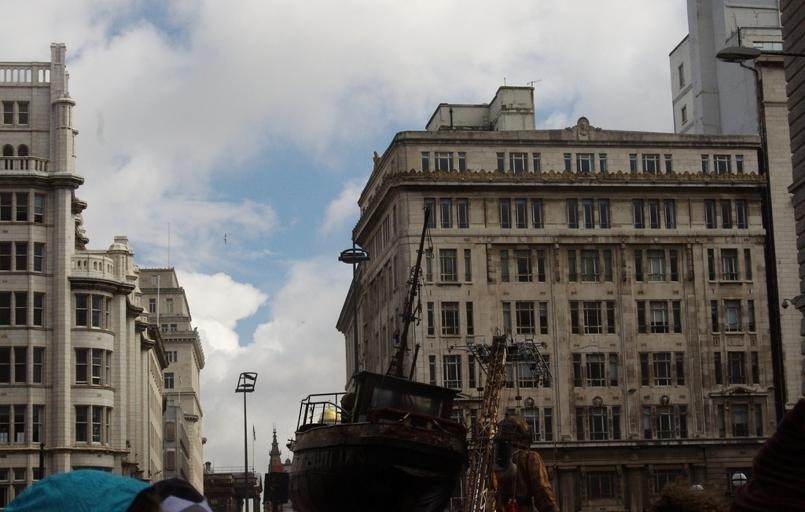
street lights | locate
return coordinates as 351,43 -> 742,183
727,469 -> 748,506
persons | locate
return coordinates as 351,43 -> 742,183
486,415 -> 561,512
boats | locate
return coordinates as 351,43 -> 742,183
289,205 -> 470,510
